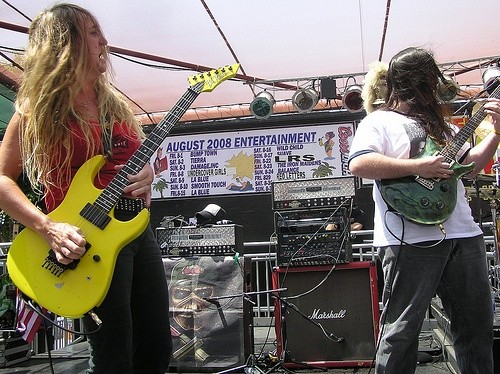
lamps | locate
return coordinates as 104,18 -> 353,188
438,73 -> 457,100
341,84 -> 367,113
481,68 -> 500,97
251,92 -> 276,120
293,89 -> 319,113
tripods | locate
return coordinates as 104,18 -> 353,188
215,287 -> 287,374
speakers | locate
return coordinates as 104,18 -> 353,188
272,261 -> 381,368
161,255 -> 254,372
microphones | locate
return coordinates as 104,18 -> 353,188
338,337 -> 345,342
203,298 -> 219,305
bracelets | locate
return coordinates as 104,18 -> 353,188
492,129 -> 500,135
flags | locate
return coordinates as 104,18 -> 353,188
16,288 -> 48,343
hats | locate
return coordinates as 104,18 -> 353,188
195,203 -> 226,220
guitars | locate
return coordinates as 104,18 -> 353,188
376,86 -> 500,228
6,61 -> 240,319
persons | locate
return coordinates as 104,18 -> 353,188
0,3 -> 173,374
325,207 -> 367,261
347,47 -> 500,374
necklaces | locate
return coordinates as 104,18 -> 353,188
77,94 -> 98,105
